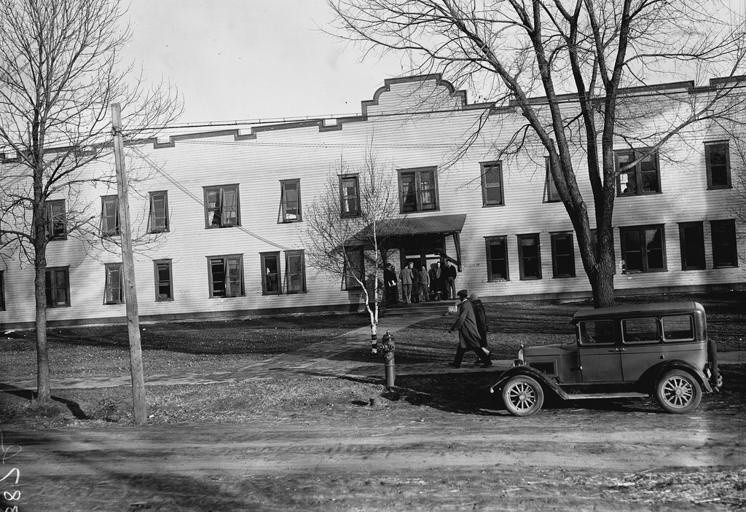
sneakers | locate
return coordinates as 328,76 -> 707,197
449,362 -> 461,369
480,361 -> 493,368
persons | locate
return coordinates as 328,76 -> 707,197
427,264 -> 437,301
435,261 -> 445,301
406,261 -> 421,303
391,265 -> 401,303
399,264 -> 415,303
443,258 -> 458,300
447,288 -> 493,369
384,263 -> 397,303
468,291 -> 493,366
418,264 -> 431,302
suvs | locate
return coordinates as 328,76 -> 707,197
487,298 -> 723,418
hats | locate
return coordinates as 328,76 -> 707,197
457,290 -> 468,296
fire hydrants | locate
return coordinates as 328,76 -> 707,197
380,330 -> 398,388
